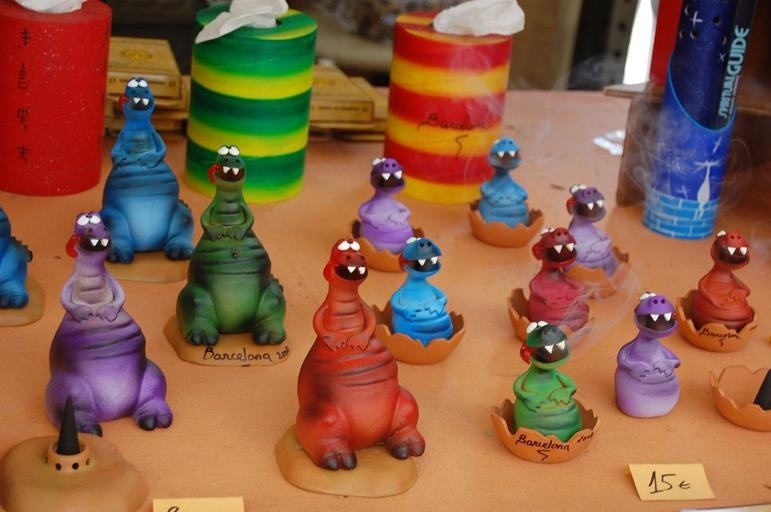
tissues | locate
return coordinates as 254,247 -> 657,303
0,0 -> 115,197
181,0 -> 319,203
381,1 -> 527,206
